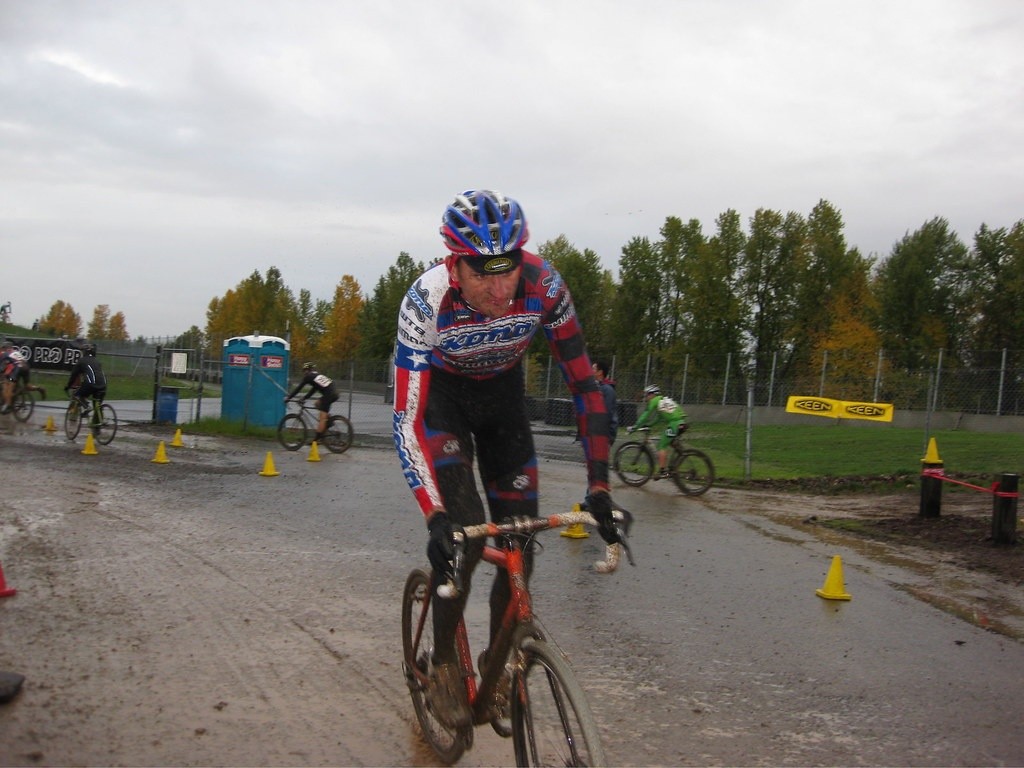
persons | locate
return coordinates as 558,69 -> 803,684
625,383 -> 692,480
388,188 -> 634,739
0,338 -> 47,414
576,361 -> 619,511
65,343 -> 107,438
283,361 -> 340,442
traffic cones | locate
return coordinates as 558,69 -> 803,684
259,451 -> 280,476
921,438 -> 943,463
151,441 -> 170,463
81,434 -> 98,454
815,555 -> 851,599
169,429 -> 184,446
306,441 -> 321,462
560,503 -> 590,538
44,416 -> 58,431
0,560 -> 16,596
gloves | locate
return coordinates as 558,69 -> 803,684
284,397 -> 290,403
627,427 -> 635,432
640,425 -> 650,432
427,511 -> 455,574
581,491 -> 633,544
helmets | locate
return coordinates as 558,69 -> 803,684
439,189 -> 530,255
303,362 -> 315,369
79,344 -> 97,352
643,384 -> 660,393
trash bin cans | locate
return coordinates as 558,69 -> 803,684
157,385 -> 177,425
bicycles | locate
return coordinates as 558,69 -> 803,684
613,425 -> 714,496
402,503 -> 637,768
277,396 -> 353,454
0,368 -> 34,422
64,385 -> 118,445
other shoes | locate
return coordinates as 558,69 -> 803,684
654,468 -> 668,480
39,389 -> 46,400
80,407 -> 92,416
425,663 -> 472,728
314,432 -> 322,441
478,649 -> 513,737
